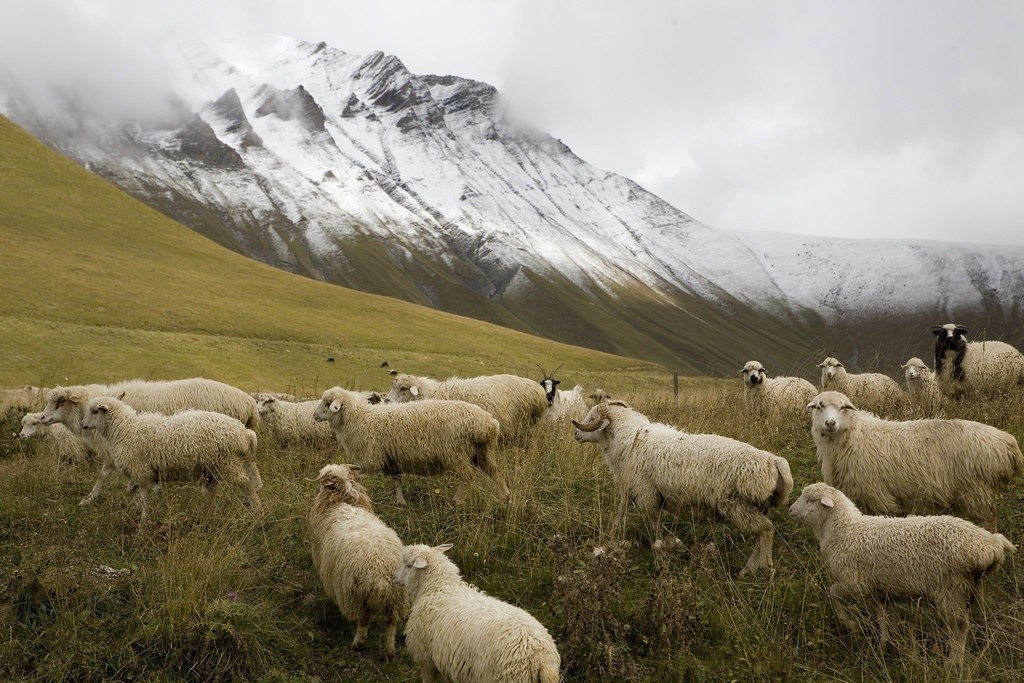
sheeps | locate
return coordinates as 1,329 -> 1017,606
571,400 -> 794,579
739,361 -> 819,423
255,390 -> 335,443
815,357 -> 904,411
19,376 -> 265,527
305,464 -> 562,683
898,357 -> 935,415
313,362 -> 613,497
806,391 -> 1024,534
788,482 -> 1019,665
928,323 -> 1024,403
